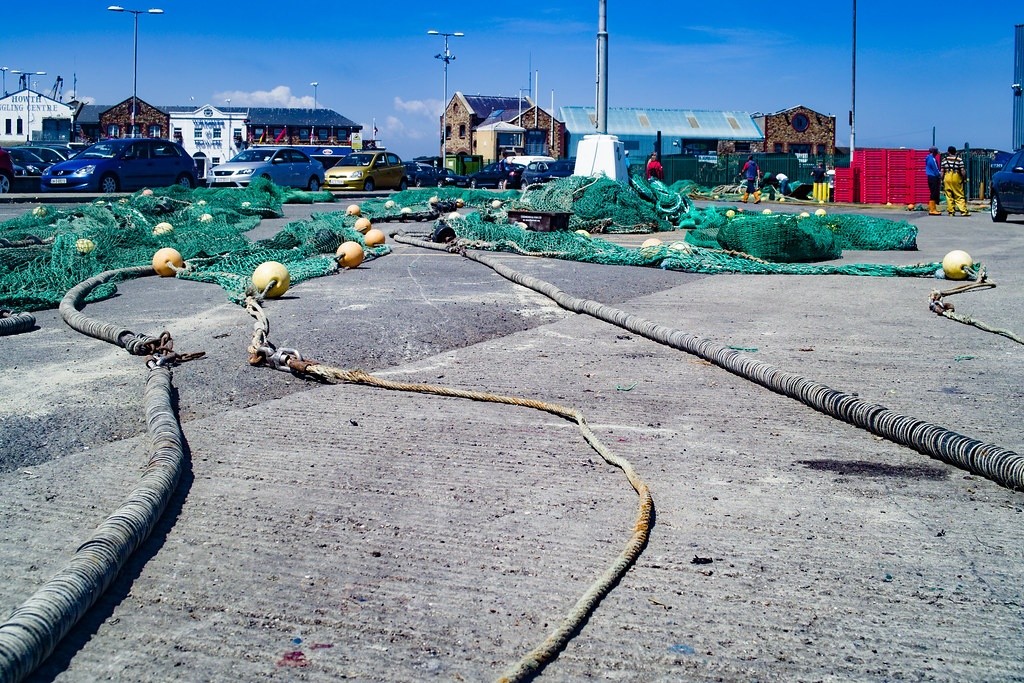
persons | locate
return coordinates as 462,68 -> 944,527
646,151 -> 664,181
624,150 -> 632,173
775,172 -> 789,197
941,146 -> 972,217
925,146 -> 941,216
496,170 -> 517,190
810,163 -> 829,183
762,172 -> 774,187
741,155 -> 761,204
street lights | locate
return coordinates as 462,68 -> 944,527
427,30 -> 465,166
11,68 -> 47,145
225,98 -> 231,162
107,5 -> 164,135
310,82 -> 319,144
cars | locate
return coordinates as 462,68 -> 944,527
415,167 -> 470,189
0,142 -> 95,194
322,150 -> 408,192
989,149 -> 1024,223
39,138 -> 199,193
205,146 -> 326,191
468,162 -> 526,191
495,156 -> 576,188
402,161 -> 432,185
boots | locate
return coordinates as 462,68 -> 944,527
742,192 -> 749,203
928,200 -> 941,215
753,192 -> 761,204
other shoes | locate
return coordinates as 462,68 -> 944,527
960,211 -> 971,216
948,212 -> 956,216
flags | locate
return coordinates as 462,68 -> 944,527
248,126 -> 352,146
374,122 -> 378,136
